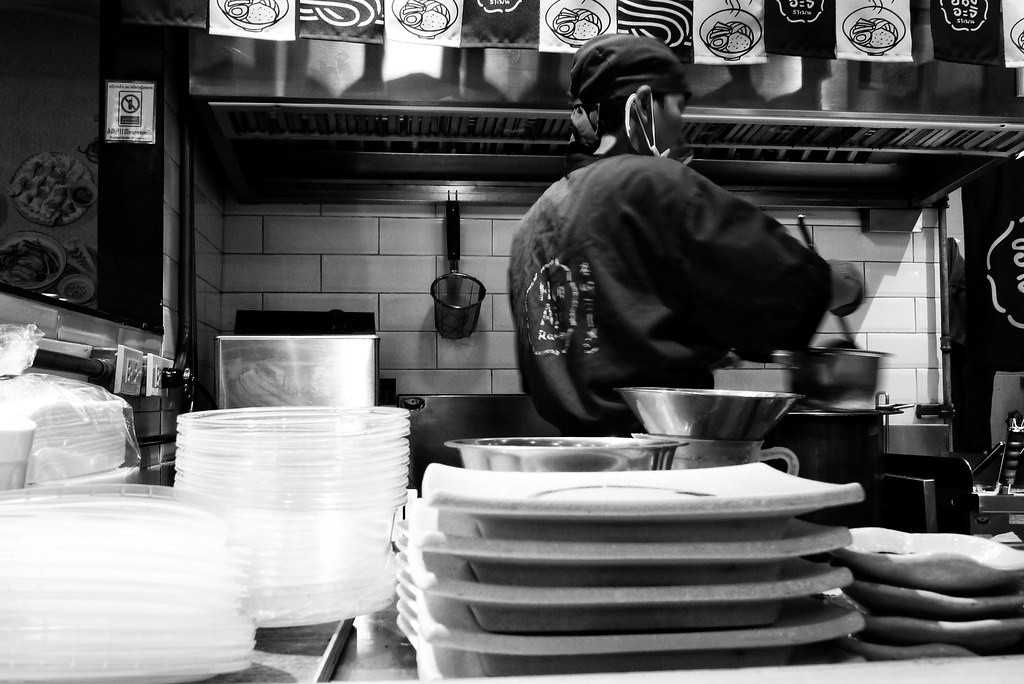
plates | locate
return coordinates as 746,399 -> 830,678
2,403 -> 410,683
392,463 -> 1024,684
7,152 -> 94,227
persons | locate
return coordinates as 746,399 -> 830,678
507,32 -> 863,439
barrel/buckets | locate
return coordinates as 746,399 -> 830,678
760,391 -> 905,528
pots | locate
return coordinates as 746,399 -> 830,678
737,343 -> 889,404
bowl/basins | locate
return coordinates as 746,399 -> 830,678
68,180 -> 98,208
442,434 -> 690,472
58,273 -> 94,303
0,229 -> 67,290
614,384 -> 805,441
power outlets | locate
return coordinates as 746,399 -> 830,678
114,344 -> 143,396
143,353 -> 173,397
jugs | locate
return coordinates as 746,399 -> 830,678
631,429 -> 799,477
